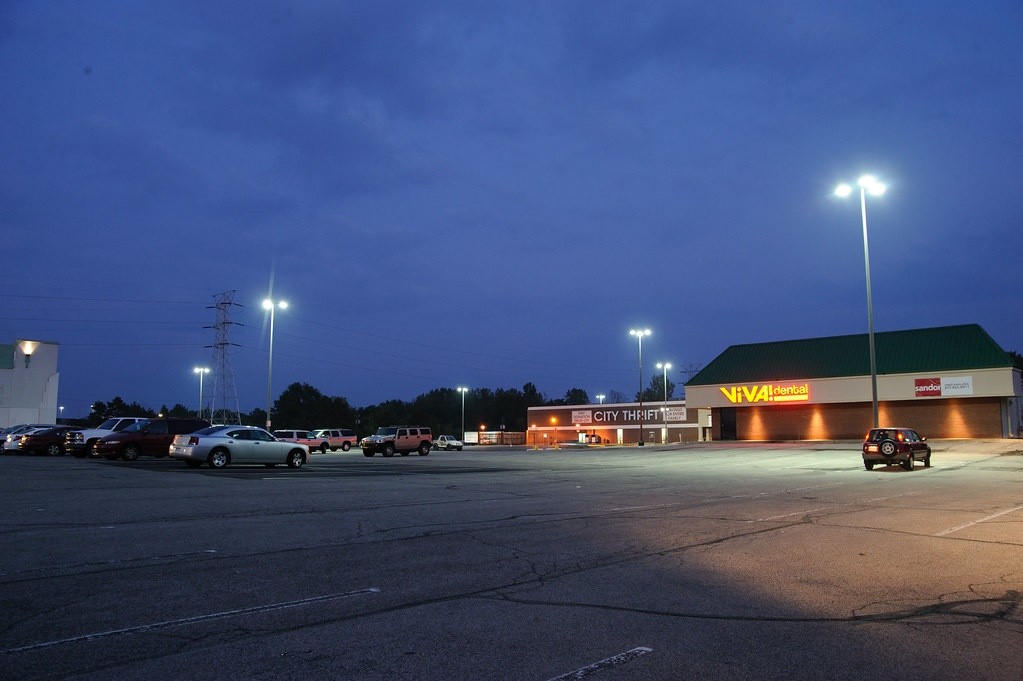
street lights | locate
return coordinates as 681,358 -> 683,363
655,362 -> 673,443
457,387 -> 469,446
193,366 -> 212,419
629,328 -> 653,448
261,298 -> 288,432
835,172 -> 887,430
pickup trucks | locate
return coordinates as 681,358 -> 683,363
432,435 -> 463,451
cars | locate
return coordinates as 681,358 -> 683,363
94,415 -> 213,461
18,425 -> 90,456
0,424 -> 52,451
169,425 -> 310,469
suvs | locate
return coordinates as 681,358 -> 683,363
359,426 -> 433,457
860,426 -> 932,470
309,429 -> 358,452
64,418 -> 148,458
275,428 -> 330,454
